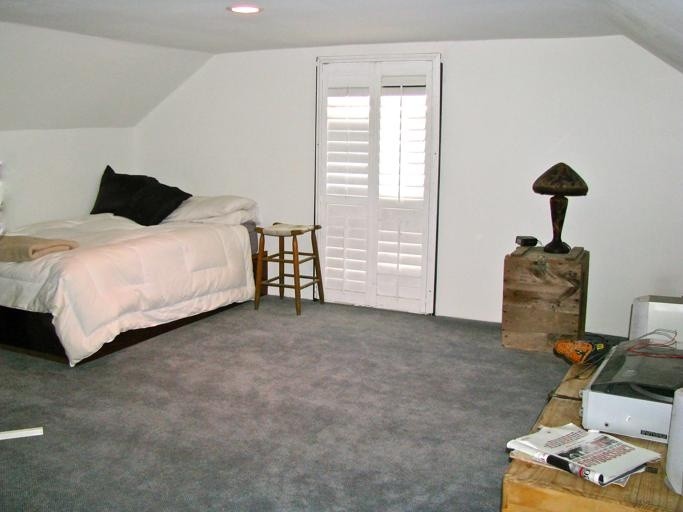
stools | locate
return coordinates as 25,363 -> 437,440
251,222 -> 325,316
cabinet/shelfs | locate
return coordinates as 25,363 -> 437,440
500,250 -> 589,354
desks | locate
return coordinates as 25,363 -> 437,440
499,362 -> 681,511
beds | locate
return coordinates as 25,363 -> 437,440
0,212 -> 269,369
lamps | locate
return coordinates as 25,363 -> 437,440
531,162 -> 588,253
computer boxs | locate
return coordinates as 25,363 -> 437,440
628,294 -> 683,350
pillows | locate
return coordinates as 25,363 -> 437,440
88,164 -> 258,227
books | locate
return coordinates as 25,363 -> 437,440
506,423 -> 661,488
